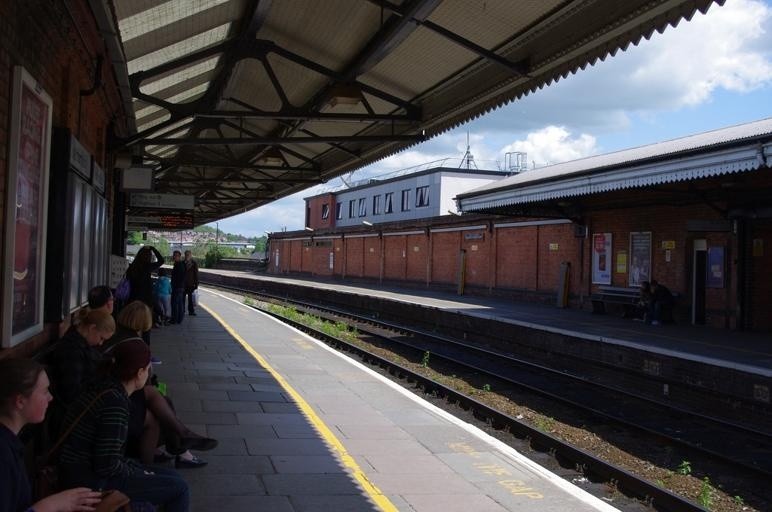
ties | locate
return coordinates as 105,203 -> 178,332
186,261 -> 189,272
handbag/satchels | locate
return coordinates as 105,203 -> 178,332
115,278 -> 130,300
94,490 -> 132,512
39,464 -> 66,499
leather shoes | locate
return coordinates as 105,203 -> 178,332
154,452 -> 173,463
166,432 -> 219,469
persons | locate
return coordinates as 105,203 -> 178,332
125,247 -> 166,365
153,249 -> 198,324
632,280 -> 676,325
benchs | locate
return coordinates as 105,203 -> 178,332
587,285 -> 681,320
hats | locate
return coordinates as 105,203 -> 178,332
116,340 -> 151,375
